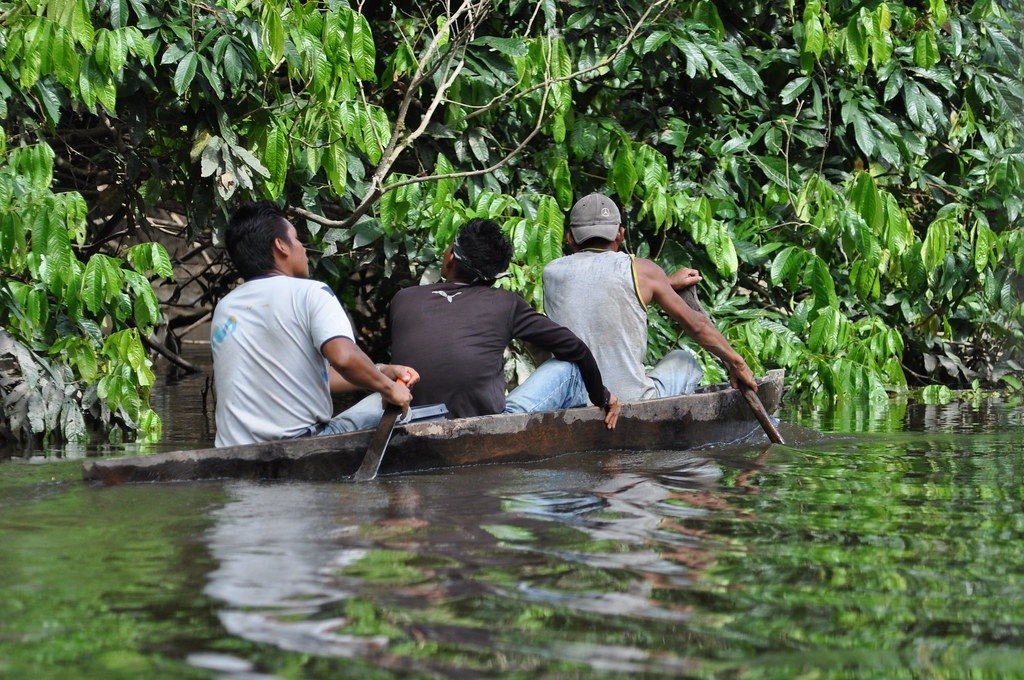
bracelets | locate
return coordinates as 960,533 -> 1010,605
374,364 -> 383,373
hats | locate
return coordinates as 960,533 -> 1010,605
569,192 -> 622,245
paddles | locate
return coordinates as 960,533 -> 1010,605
351,378 -> 410,484
679,283 -> 788,446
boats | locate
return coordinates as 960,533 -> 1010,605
83,365 -> 783,484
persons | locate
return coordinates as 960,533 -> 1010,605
542,193 -> 758,404
387,219 -> 622,429
210,209 -> 419,449
